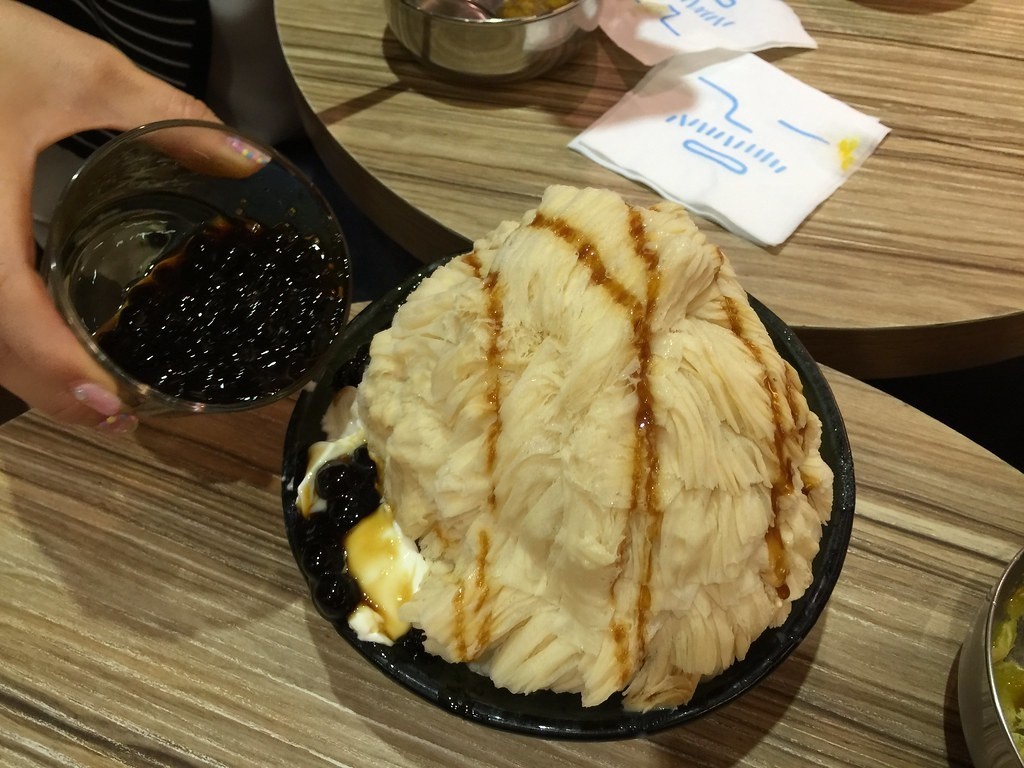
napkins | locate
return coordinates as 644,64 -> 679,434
566,47 -> 893,250
592,0 -> 818,68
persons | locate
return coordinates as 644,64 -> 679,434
0,0 -> 268,444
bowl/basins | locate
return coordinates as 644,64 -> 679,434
958,547 -> 1024,767
386,0 -> 583,81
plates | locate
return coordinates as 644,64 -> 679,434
282,258 -> 857,740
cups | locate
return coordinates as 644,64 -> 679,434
38,119 -> 352,419
